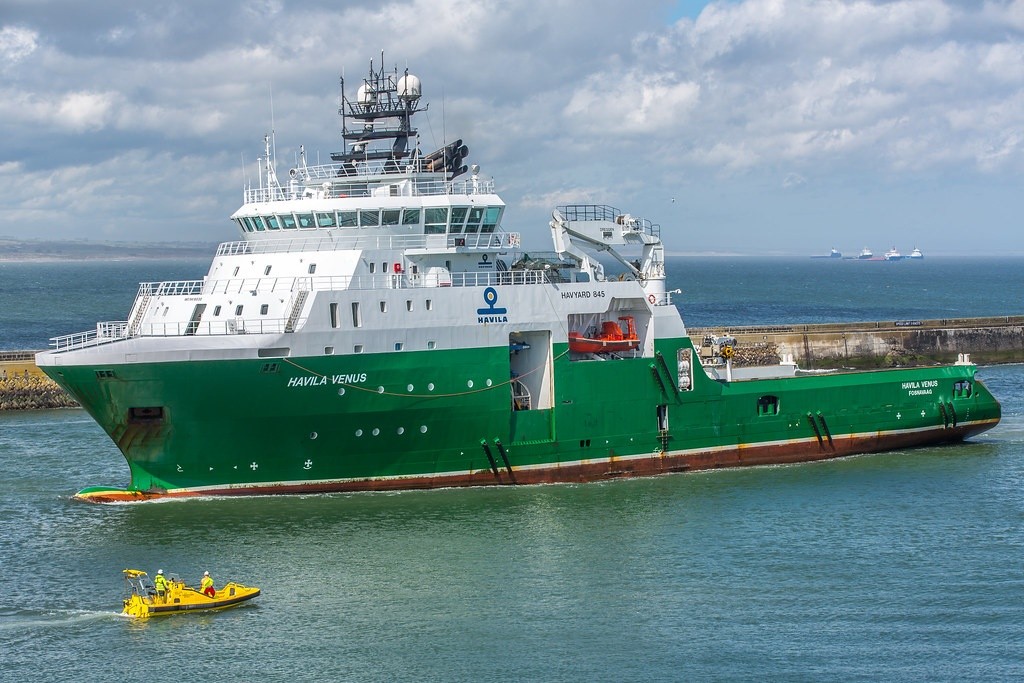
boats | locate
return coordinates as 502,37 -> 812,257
122,567 -> 262,616
858,246 -> 873,258
911,244 -> 923,258
569,315 -> 641,354
33,46 -> 1003,506
830,247 -> 842,257
884,246 -> 901,260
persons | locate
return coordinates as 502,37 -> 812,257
155,570 -> 169,604
199,571 -> 215,597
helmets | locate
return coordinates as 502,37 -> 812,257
204,571 -> 209,576
158,570 -> 163,574
